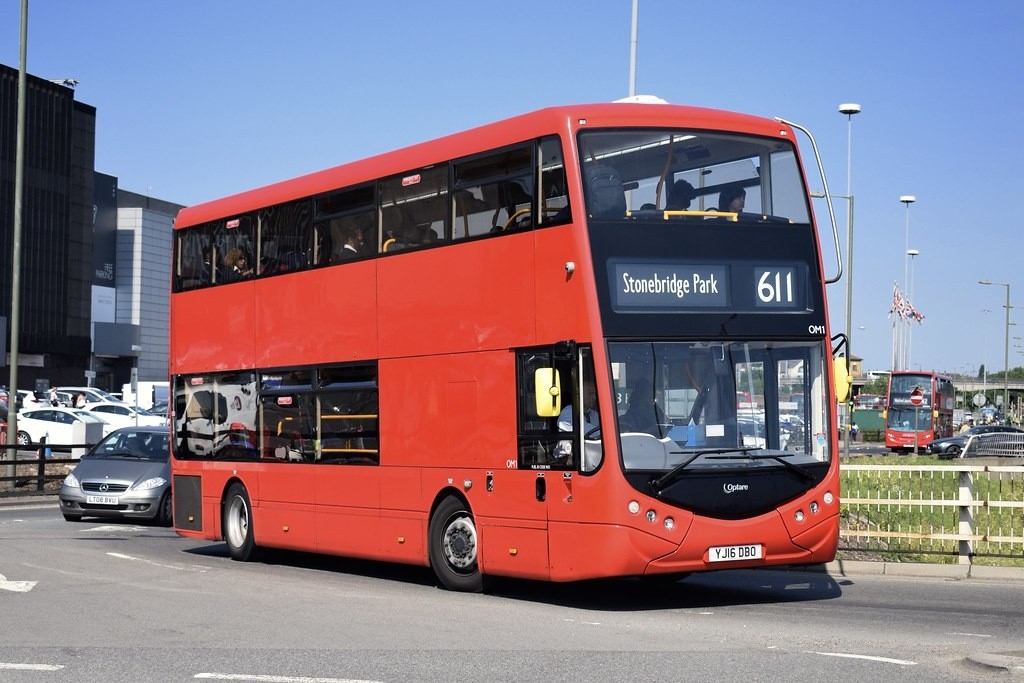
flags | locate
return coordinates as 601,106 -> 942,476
888,284 -> 925,327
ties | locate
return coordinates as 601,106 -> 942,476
585,413 -> 590,424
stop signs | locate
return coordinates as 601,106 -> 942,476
910,391 -> 923,406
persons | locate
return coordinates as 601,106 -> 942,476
937,426 -> 944,439
336,232 -> 365,264
553,381 -> 600,458
980,416 -> 999,421
852,422 -> 860,442
997,403 -> 1001,412
715,186 -> 746,221
196,246 -> 253,286
229,422 -> 259,461
71,391 -> 86,409
625,377 -> 665,439
50,387 -> 61,407
664,180 -> 696,211
1,391 -> 10,418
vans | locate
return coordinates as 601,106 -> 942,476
952,408 -> 1006,431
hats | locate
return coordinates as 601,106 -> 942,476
230,421 -> 246,430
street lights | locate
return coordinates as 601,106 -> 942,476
859,326 -> 865,378
837,103 -> 862,465
978,280 -> 1010,422
898,195 -> 919,391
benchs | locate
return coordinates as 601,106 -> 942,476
383,239 -> 421,252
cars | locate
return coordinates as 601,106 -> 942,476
0,382 -> 170,450
59,426 -> 173,527
925,425 -> 1024,460
736,391 -> 810,451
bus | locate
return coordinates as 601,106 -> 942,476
168,94 -> 853,593
854,394 -> 887,409
882,371 -> 955,456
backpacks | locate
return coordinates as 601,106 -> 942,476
585,163 -> 627,218
